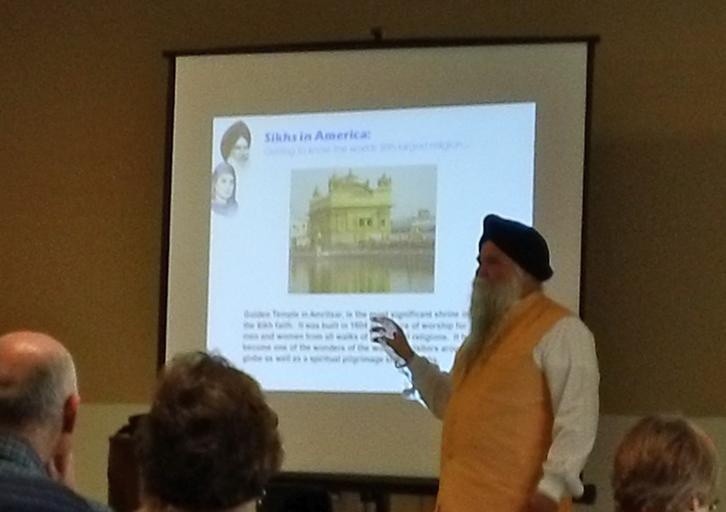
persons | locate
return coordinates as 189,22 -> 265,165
127,349 -> 286,512
608,413 -> 722,512
0,328 -> 117,512
219,121 -> 251,184
370,211 -> 601,511
209,162 -> 239,219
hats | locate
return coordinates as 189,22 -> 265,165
479,215 -> 553,280
219,121 -> 251,162
